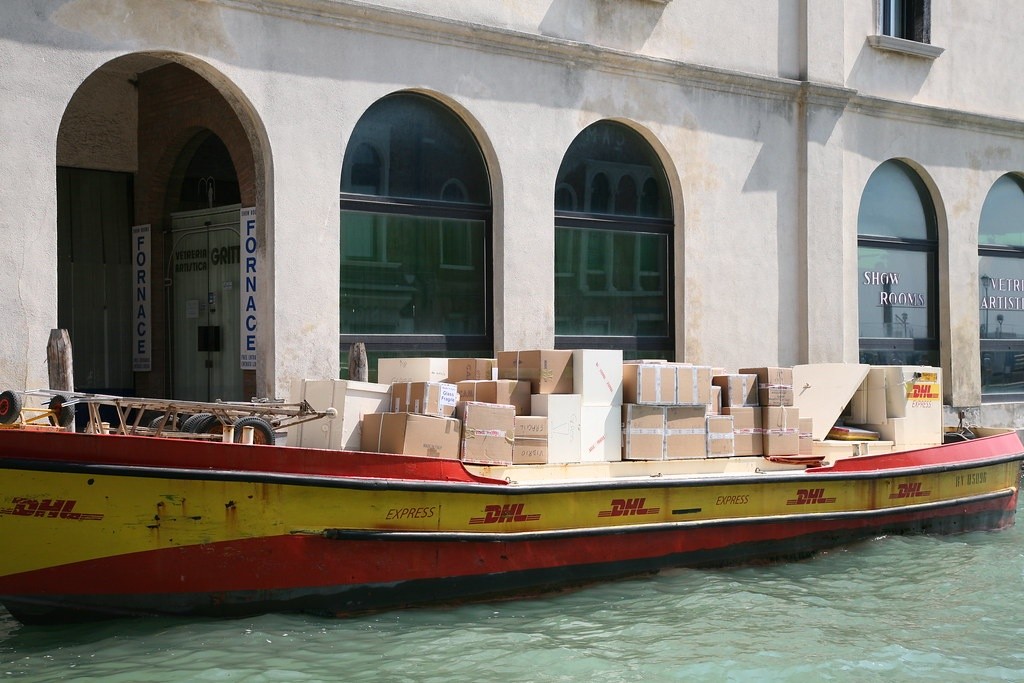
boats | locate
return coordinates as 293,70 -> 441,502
0,386 -> 1023,620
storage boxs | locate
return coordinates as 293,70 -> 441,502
762,407 -> 799,456
456,378 -> 531,417
447,359 -> 491,384
498,350 -> 574,395
360,413 -> 462,461
623,364 -> 676,406
456,401 -> 515,466
620,403 -> 665,461
511,416 -> 547,465
711,367 -> 727,387
664,404 -> 706,461
722,407 -> 763,457
739,367 -> 793,407
706,386 -> 723,417
706,414 -> 734,458
798,417 -> 813,455
391,383 -> 457,419
676,366 -> 711,406
714,374 -> 758,408
